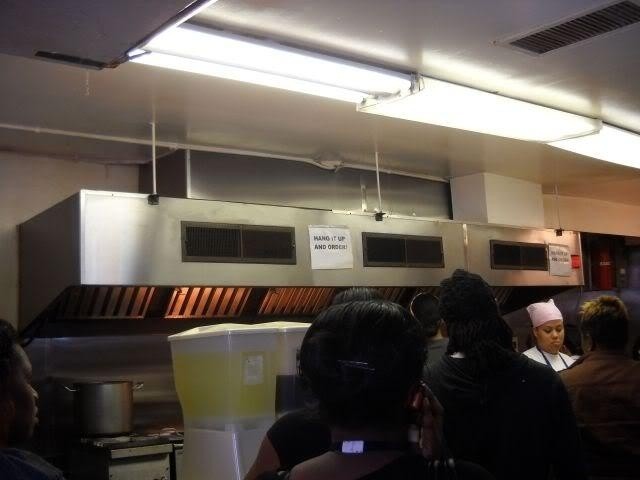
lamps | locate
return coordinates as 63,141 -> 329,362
129,22 -> 421,113
547,126 -> 640,170
356,76 -> 601,148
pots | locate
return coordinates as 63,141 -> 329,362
61,379 -> 145,437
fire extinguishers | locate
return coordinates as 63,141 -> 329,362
598,245 -> 613,289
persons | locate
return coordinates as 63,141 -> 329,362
245,267 -> 640,479
1,319 -> 68,480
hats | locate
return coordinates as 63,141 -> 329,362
526,299 -> 563,328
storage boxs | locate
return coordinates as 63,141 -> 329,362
168,320 -> 328,480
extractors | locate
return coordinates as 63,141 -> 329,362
16,185 -> 584,338
